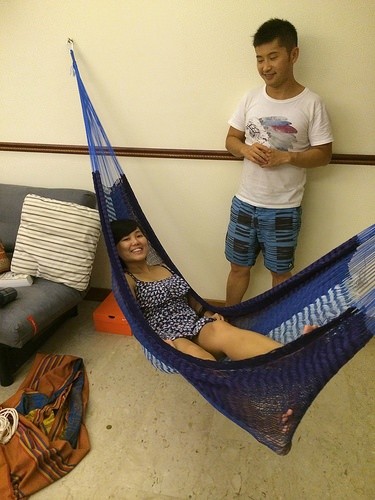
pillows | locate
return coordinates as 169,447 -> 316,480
10,194 -> 103,291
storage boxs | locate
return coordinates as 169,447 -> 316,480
93,289 -> 133,337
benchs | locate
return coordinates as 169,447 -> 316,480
0,184 -> 95,386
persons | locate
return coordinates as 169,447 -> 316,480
109,220 -> 321,454
225,17 -> 334,306
